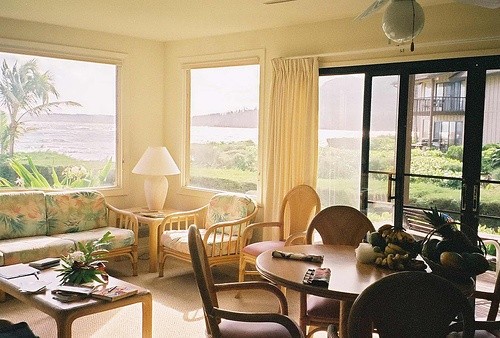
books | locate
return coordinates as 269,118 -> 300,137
51,286 -> 92,296
29,258 -> 60,270
91,285 -> 138,303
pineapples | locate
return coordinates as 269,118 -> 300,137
423,206 -> 473,252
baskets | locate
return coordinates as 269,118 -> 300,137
419,222 -> 488,278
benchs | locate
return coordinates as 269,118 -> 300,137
0,189 -> 139,300
402,206 -> 500,277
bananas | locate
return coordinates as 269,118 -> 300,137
375,224 -> 417,272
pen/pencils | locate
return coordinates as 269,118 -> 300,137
34,272 -> 40,281
108,285 -> 117,292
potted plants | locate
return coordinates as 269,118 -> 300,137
56,228 -> 114,285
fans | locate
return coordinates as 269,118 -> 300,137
351,0 -> 500,23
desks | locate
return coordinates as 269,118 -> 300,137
255,243 -> 478,338
0,258 -> 153,338
117,208 -> 197,272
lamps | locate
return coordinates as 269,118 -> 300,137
381,0 -> 425,46
132,147 -> 181,211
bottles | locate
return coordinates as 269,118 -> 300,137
356,242 -> 375,263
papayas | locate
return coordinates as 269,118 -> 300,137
440,252 -> 468,272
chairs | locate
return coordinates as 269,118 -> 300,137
301,205 -> 375,338
348,271 -> 475,338
445,271 -> 500,338
235,183 -> 321,313
188,224 -> 303,338
158,190 -> 258,277
